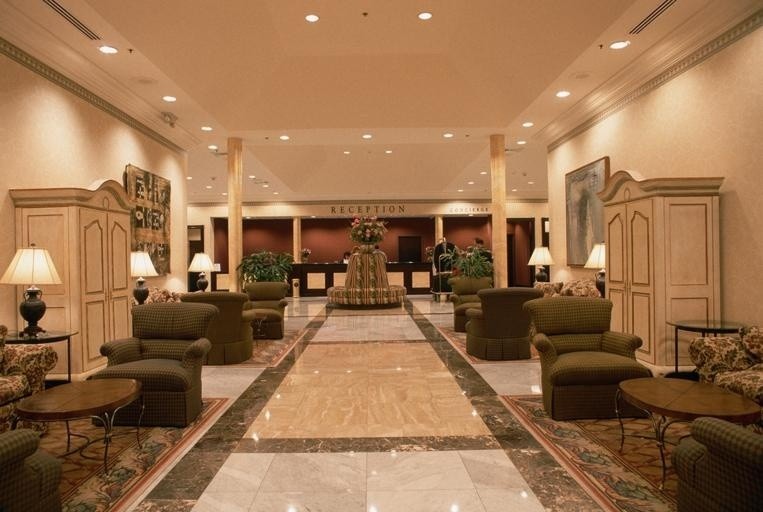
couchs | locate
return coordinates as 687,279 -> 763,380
178,281 -> 290,365
325,252 -> 407,310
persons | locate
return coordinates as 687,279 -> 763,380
340,251 -> 351,265
473,237 -> 484,250
372,245 -> 387,262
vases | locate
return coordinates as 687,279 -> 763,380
360,242 -> 375,254
303,257 -> 308,264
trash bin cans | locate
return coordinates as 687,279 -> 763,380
292,278 -> 301,297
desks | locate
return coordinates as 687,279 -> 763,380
0,330 -> 79,388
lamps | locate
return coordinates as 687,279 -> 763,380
527,246 -> 554,281
0,243 -> 62,336
130,251 -> 159,304
583,243 -> 605,298
186,252 -> 215,292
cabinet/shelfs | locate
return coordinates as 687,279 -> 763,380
7,180 -> 135,383
595,168 -> 725,379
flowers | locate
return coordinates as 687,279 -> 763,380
345,211 -> 390,245
299,247 -> 311,256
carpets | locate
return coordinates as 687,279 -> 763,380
37,398 -> 230,512
432,322 -> 540,364
